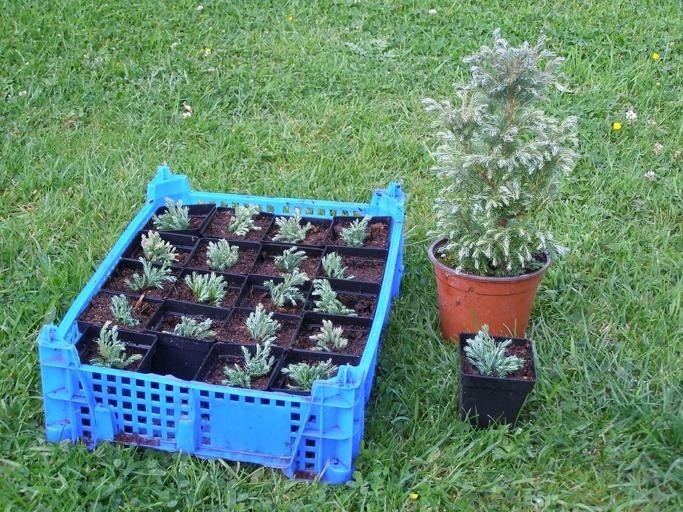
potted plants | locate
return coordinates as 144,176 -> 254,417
37,161 -> 406,487
414,31 -> 579,348
458,321 -> 539,432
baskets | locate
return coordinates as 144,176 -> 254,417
36,162 -> 406,484
